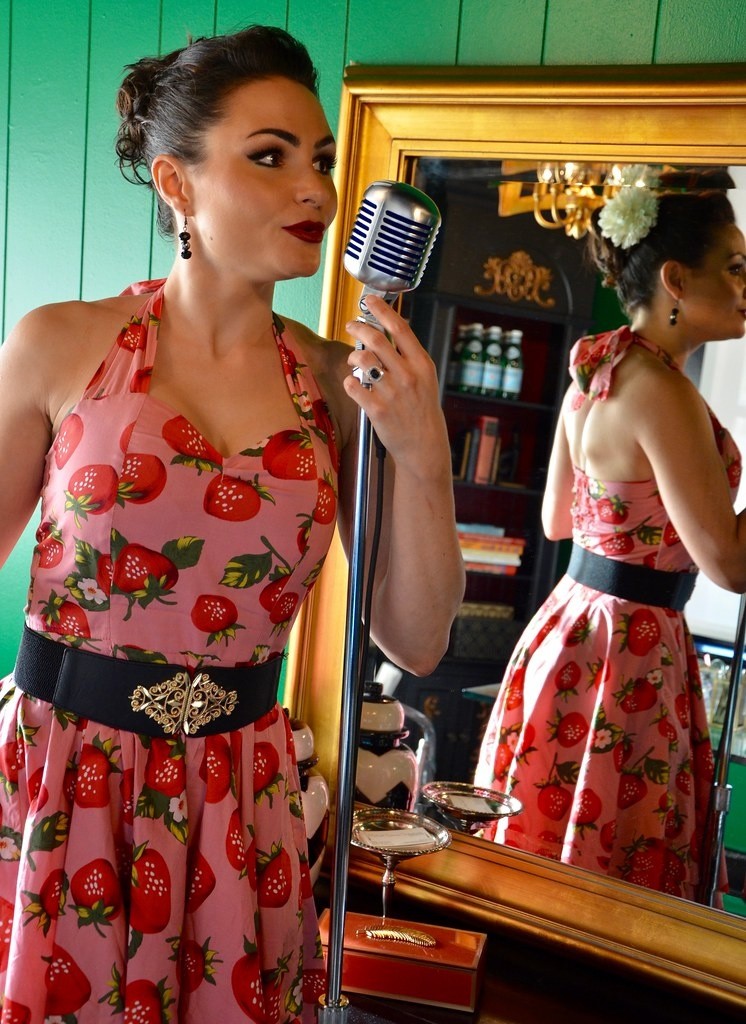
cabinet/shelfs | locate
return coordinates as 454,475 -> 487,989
393,292 -> 597,776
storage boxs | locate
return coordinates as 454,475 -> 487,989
316,909 -> 487,1013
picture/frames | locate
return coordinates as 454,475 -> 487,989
286,68 -> 746,1003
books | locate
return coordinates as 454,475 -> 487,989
458,524 -> 526,576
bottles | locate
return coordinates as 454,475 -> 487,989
280,714 -> 332,888
448,322 -> 526,399
354,681 -> 417,814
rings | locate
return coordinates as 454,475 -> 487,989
365,366 -> 387,382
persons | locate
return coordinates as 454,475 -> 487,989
474,172 -> 746,915
0,25 -> 466,1024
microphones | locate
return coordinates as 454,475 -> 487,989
344,180 -> 441,389
